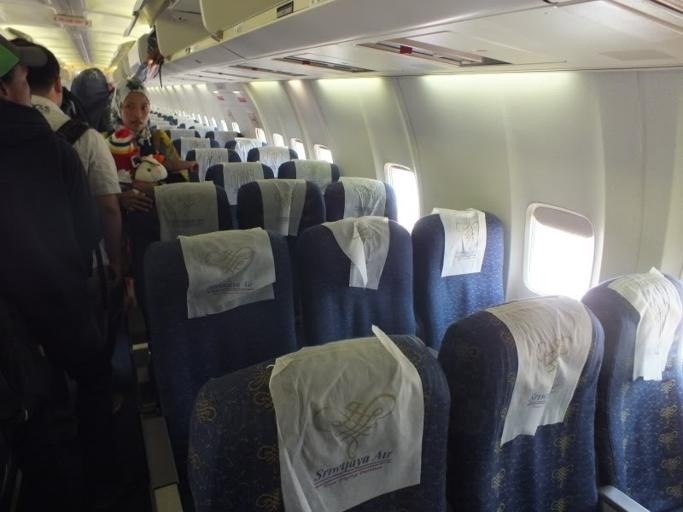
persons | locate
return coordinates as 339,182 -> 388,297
105,73 -> 200,240
65,62 -> 125,286
0,35 -> 131,296
0,45 -> 90,512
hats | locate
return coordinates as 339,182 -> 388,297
115,78 -> 150,113
0,34 -> 47,86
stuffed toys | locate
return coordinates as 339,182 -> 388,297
130,151 -> 170,199
107,124 -> 138,191
